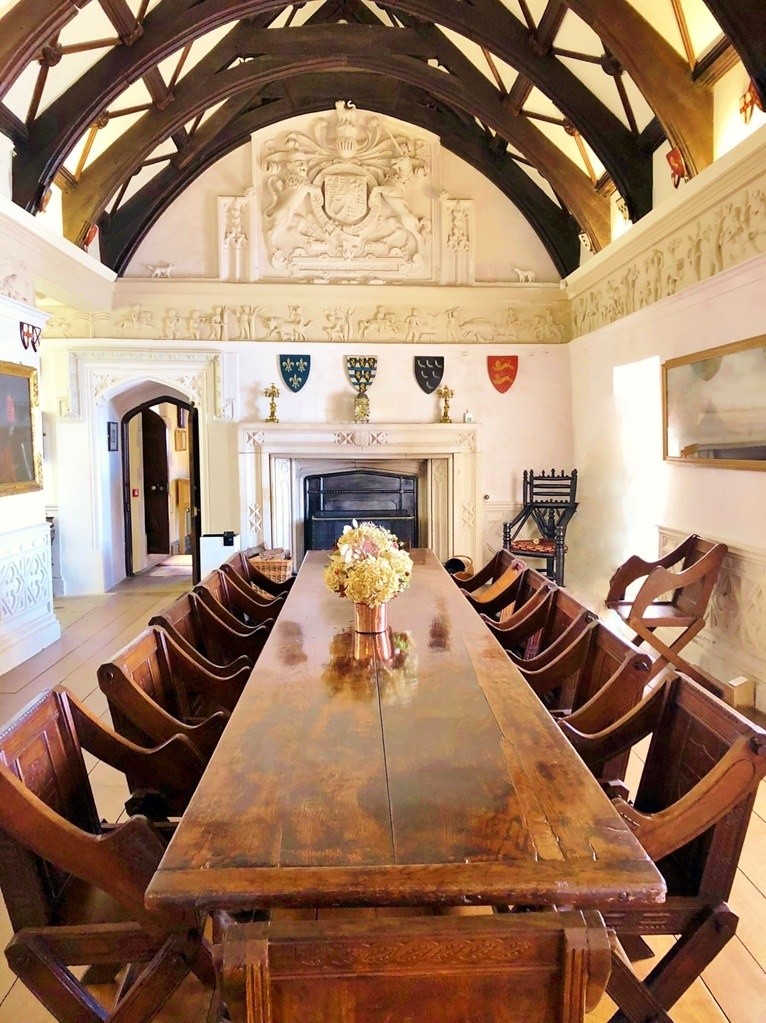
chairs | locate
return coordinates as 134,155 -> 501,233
1,684 -> 214,1023
505,465 -> 578,587
602,534 -> 724,695
193,568 -> 286,635
485,588 -> 599,707
452,547 -> 525,603
147,590 -> 269,711
496,672 -> 766,1023
218,551 -> 298,604
463,568 -> 558,658
513,618 -> 652,799
222,905 -> 617,1023
97,623 -> 251,822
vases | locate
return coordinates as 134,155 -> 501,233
353,599 -> 387,633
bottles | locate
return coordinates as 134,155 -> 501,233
464,409 -> 472,422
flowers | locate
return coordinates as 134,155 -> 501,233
325,517 -> 411,609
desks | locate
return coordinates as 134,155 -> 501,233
147,546 -> 666,911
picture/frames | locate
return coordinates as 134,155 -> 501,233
661,333 -> 766,471
0,359 -> 44,496
